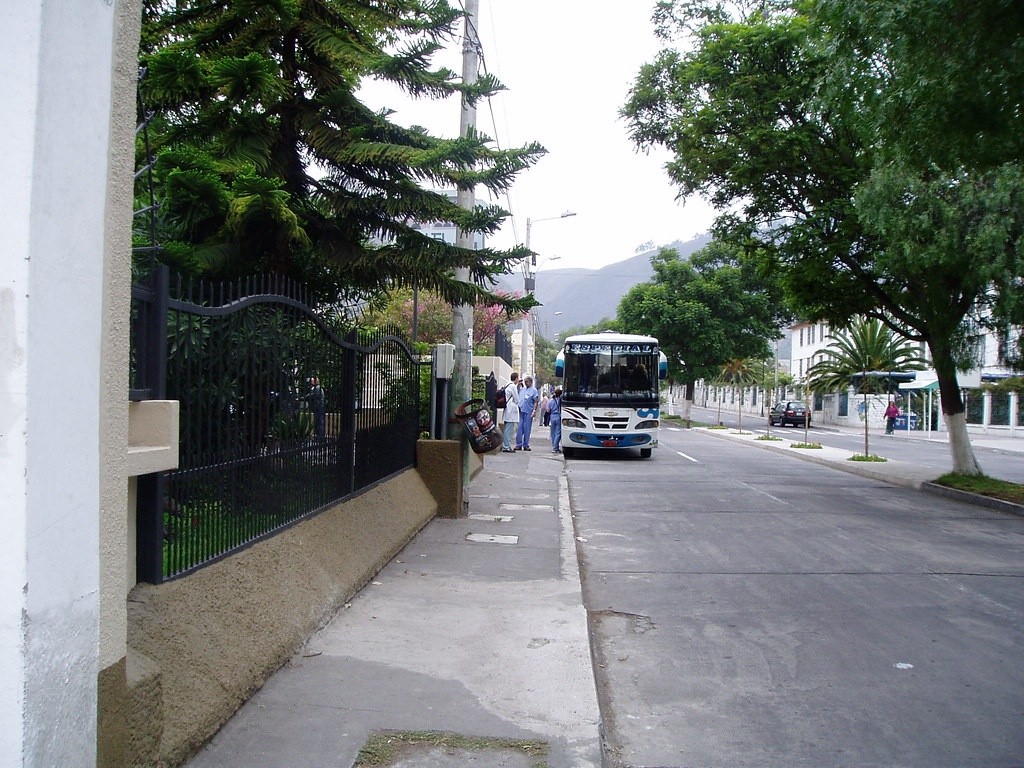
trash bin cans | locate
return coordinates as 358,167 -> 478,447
454,398 -> 504,454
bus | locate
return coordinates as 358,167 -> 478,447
555,328 -> 668,459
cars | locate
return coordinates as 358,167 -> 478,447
770,401 -> 812,428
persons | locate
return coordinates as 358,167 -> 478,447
883,400 -> 900,435
513,376 -> 540,451
501,373 -> 522,454
566,354 -> 655,393
539,391 -> 556,427
545,389 -> 563,454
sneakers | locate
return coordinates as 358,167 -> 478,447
524,447 -> 531,451
514,447 -> 522,450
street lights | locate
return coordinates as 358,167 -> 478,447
532,256 -> 560,379
546,311 -> 562,339
521,211 -> 576,382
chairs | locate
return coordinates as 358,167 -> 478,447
592,363 -> 649,391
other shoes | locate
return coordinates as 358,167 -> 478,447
539,424 -> 543,426
503,449 -> 515,453
891,431 -> 894,434
885,432 -> 888,434
552,450 -> 561,453
312,437 -> 327,443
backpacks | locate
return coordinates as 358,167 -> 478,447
493,383 -> 513,408
